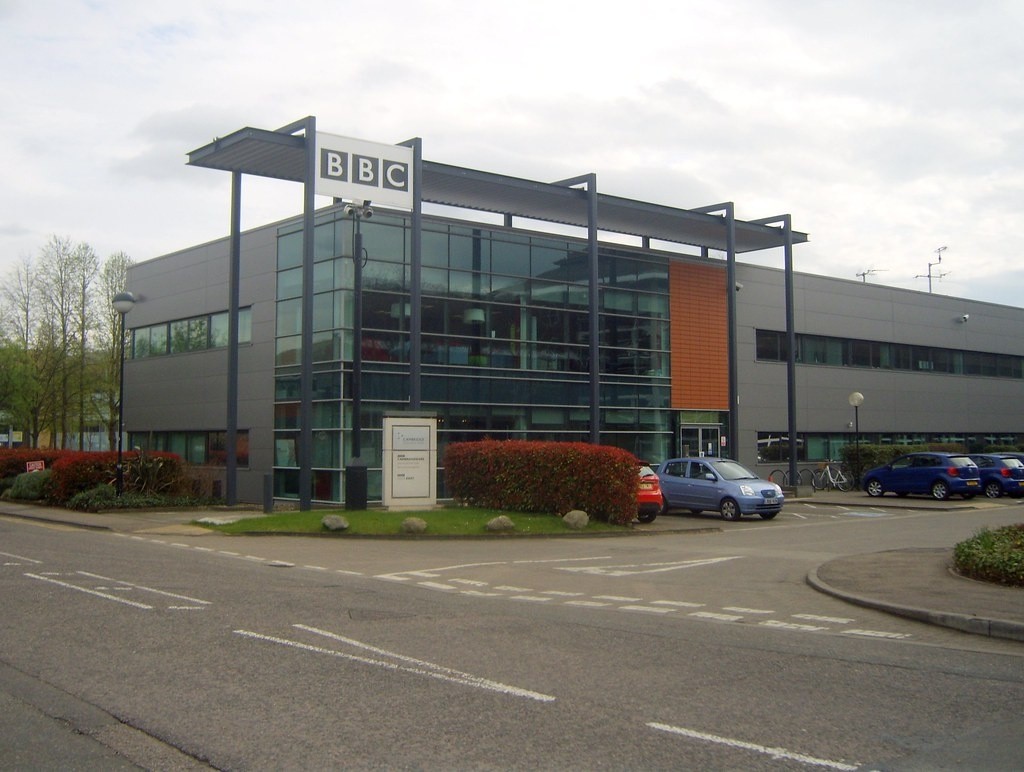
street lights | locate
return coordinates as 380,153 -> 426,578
848,392 -> 865,461
112,290 -> 138,510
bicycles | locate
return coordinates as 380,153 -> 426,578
812,461 -> 857,492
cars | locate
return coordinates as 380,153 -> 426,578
638,461 -> 664,524
860,452 -> 982,501
656,458 -> 786,521
964,452 -> 1024,499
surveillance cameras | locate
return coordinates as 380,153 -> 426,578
963,314 -> 970,320
734,281 -> 743,291
